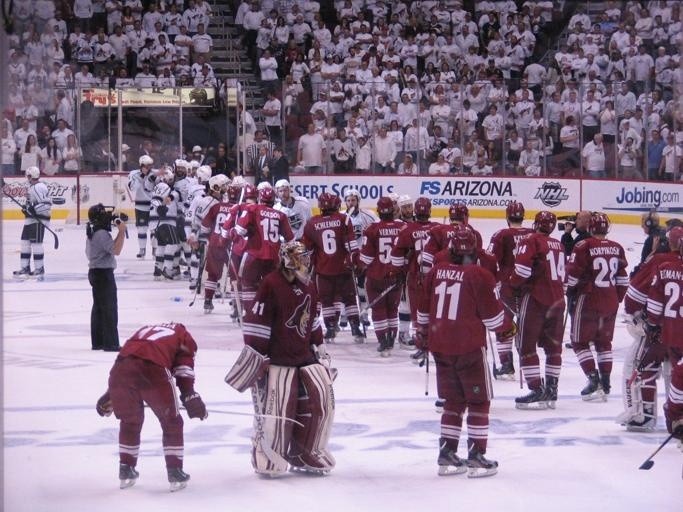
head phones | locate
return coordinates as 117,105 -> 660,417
644,209 -> 656,227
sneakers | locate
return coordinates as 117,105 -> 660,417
214,287 -> 221,297
435,398 -> 447,406
204,300 -> 214,309
13,266 -> 29,275
410,349 -> 426,359
136,250 -> 200,289
119,463 -> 139,479
26,266 -> 44,275
167,466 -> 190,482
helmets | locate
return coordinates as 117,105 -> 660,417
318,193 -> 341,209
26,167 -> 41,179
138,146 -> 290,204
449,203 -> 468,218
344,189 -> 362,202
452,229 -> 479,255
278,241 -> 314,286
590,212 -> 610,233
506,203 -> 525,217
535,211 -> 556,233
666,225 -> 683,243
377,192 -> 432,215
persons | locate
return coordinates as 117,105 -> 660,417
392,199 -> 438,349
234,0 -> 683,183
414,229 -> 517,477
358,196 -> 407,355
486,203 -> 533,382
397,197 -> 414,349
230,188 -> 294,325
145,159 -> 270,312
85,203 -> 127,352
623,214 -> 683,438
340,189 -> 375,330
410,203 -> 482,362
565,212 -> 630,403
304,192 -> 365,343
222,184 -> 258,323
274,179 -> 311,241
509,211 -> 567,411
559,210 -> 592,348
0,0 -> 234,177
96,322 -> 209,491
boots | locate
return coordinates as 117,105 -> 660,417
629,404 -> 654,425
581,370 -> 610,396
515,378 -> 557,403
377,331 -> 415,351
323,314 -> 370,338
465,438 -> 498,469
437,437 -> 468,467
496,351 -> 515,375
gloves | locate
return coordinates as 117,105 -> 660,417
96,392 -> 113,416
180,392 -> 208,420
